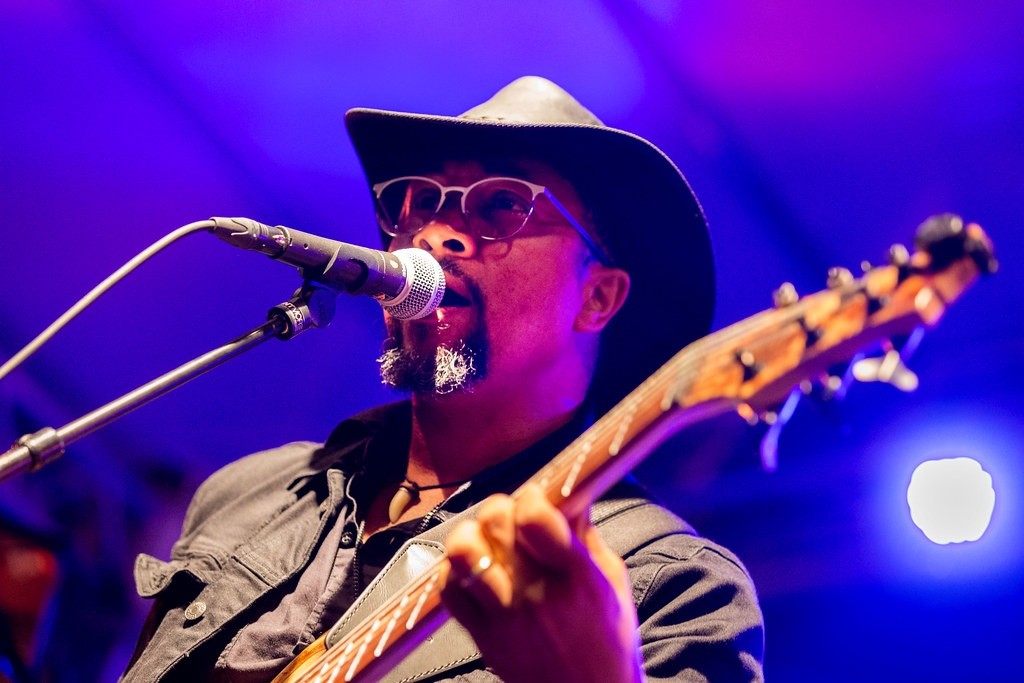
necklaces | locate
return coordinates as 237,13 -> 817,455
389,478 -> 468,524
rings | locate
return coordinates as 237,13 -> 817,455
470,555 -> 497,578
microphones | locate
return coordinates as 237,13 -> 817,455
209,216 -> 447,322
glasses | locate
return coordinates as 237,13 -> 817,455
373,175 -> 612,267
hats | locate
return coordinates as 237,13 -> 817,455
345,75 -> 713,421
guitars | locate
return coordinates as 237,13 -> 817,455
268,212 -> 999,683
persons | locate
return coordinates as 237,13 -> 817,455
119,76 -> 765,683
